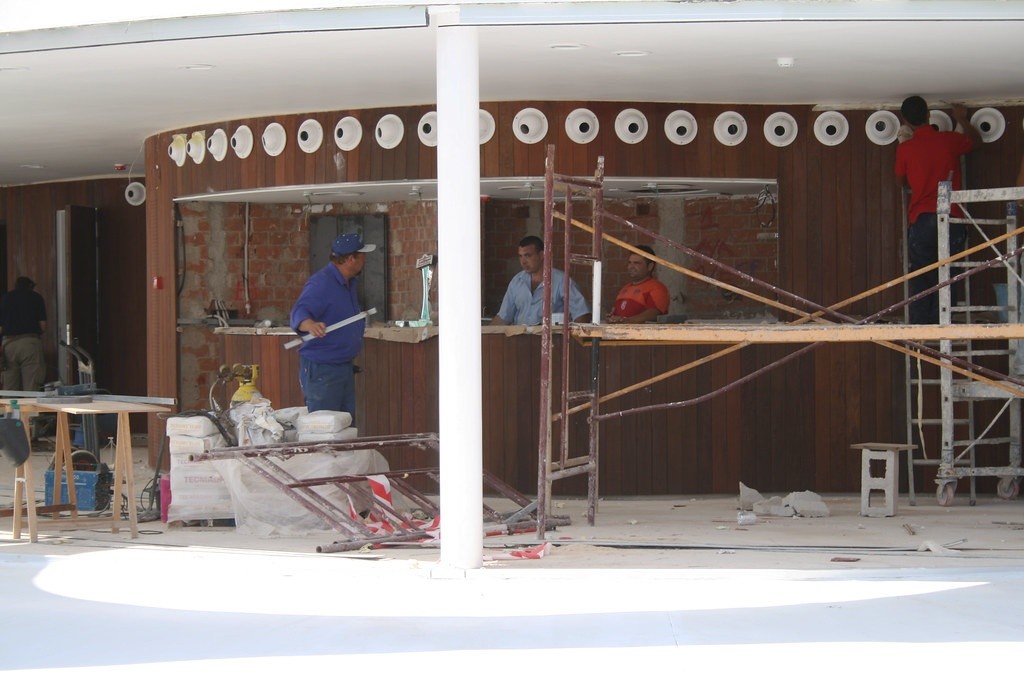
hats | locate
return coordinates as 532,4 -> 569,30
330,232 -> 377,254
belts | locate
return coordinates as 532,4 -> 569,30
2,335 -> 40,339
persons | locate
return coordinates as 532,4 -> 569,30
491,236 -> 591,324
290,233 -> 376,426
605,244 -> 670,323
896,95 -> 980,320
1,277 -> 49,428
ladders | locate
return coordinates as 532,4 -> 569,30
900,151 -> 976,508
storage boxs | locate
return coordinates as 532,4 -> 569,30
44,450 -> 111,510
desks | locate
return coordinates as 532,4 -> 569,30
1,400 -> 172,543
